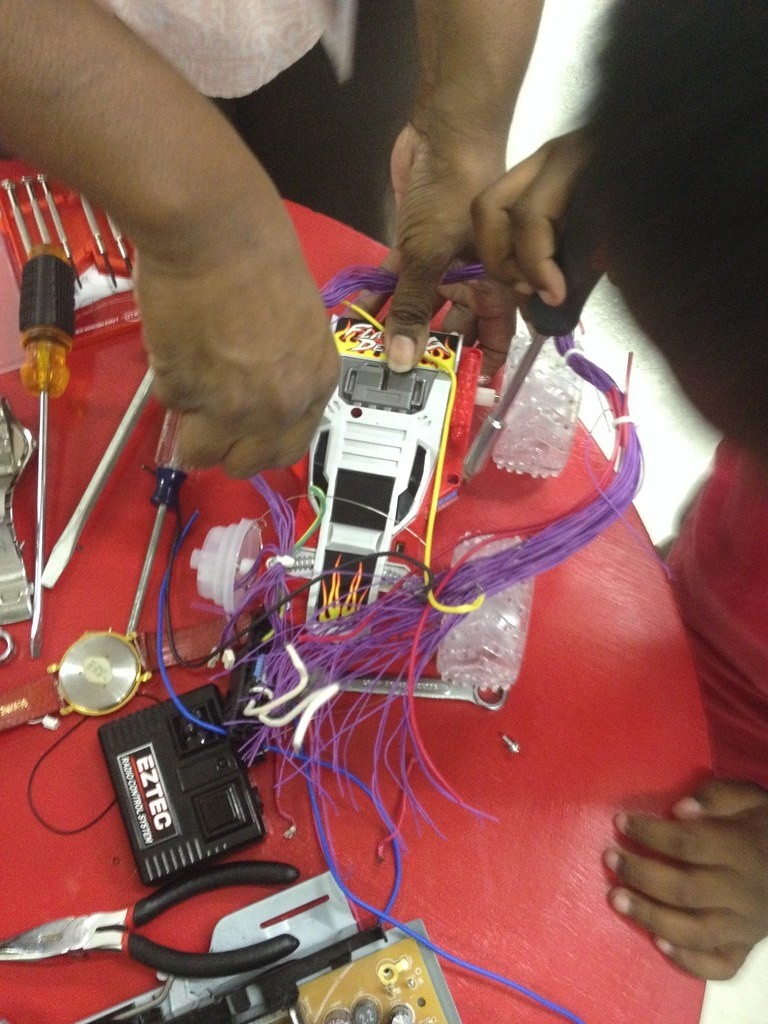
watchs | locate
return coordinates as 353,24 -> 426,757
2,614 -> 259,721
1,394 -> 37,624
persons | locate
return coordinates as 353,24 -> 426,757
472,0 -> 767,981
0,1 -> 543,481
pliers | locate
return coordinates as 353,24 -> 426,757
1,859 -> 302,1007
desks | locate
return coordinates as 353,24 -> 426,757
3,153 -> 709,1024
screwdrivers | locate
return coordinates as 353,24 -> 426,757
123,407 -> 197,645
40,363 -> 159,591
459,233 -> 607,485
16,246 -> 79,661
